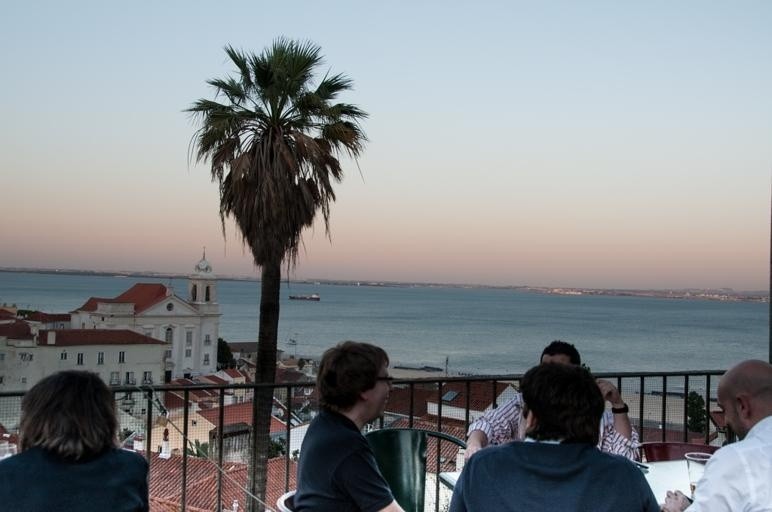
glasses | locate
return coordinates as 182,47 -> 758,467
376,377 -> 393,384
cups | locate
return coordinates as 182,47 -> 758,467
684,451 -> 712,502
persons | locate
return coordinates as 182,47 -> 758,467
0,370 -> 149,512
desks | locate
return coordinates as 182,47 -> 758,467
439,462 -> 704,510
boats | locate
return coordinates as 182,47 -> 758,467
288,292 -> 321,302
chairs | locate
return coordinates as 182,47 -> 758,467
635,441 -> 724,463
276,427 -> 466,511
